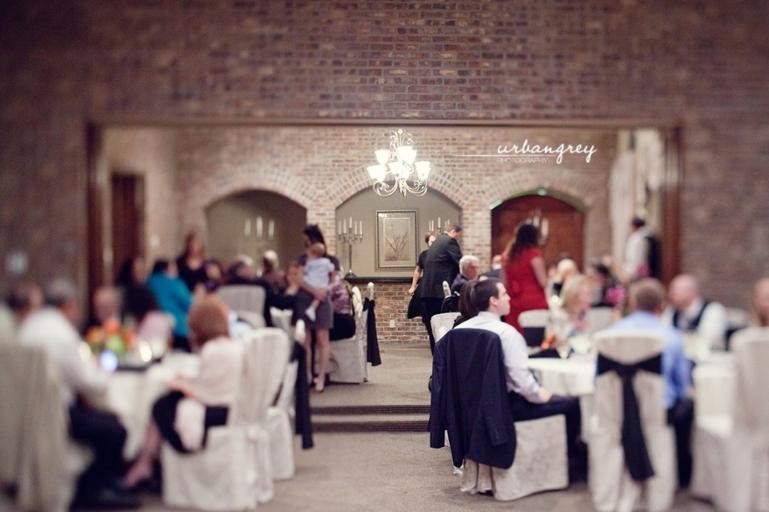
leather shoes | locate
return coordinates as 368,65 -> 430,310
96,488 -> 138,509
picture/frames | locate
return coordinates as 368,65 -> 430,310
372,207 -> 421,273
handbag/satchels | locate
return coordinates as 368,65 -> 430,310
328,312 -> 356,342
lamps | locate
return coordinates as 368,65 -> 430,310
365,129 -> 433,199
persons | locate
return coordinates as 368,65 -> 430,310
452,279 -> 586,470
1,216 -> 769,511
501,223 -> 550,337
419,222 -> 464,355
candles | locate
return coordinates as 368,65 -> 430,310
429,217 -> 452,234
526,217 -> 550,241
336,217 -> 364,237
244,217 -> 275,240
